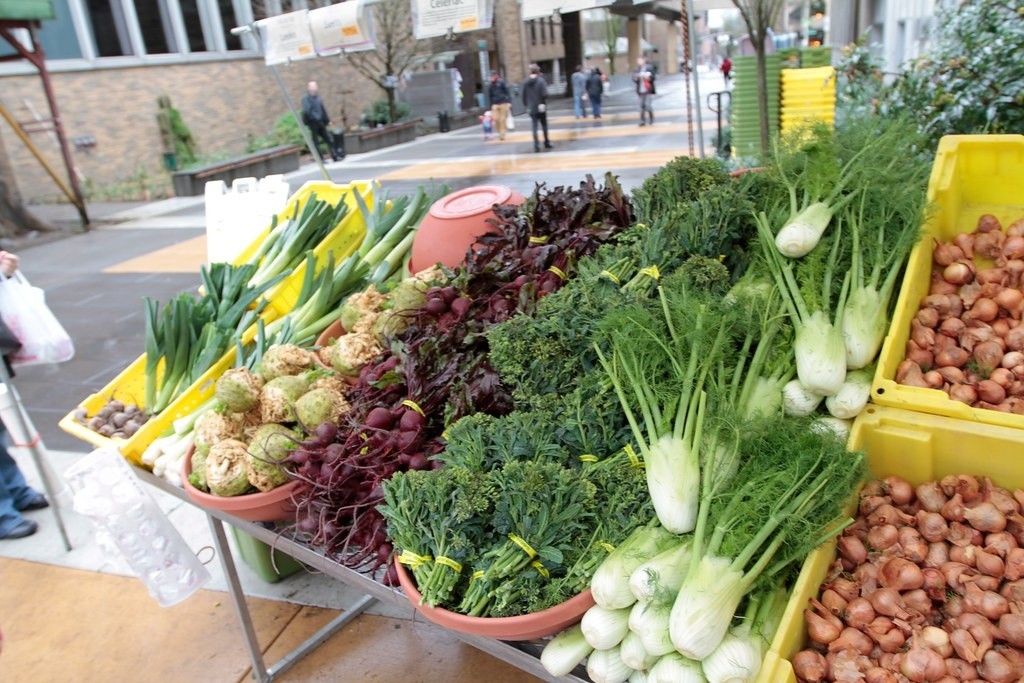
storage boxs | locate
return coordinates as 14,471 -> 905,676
870,133 -> 1024,430
59,309 -> 259,472
728,46 -> 835,158
198,180 -> 392,321
756,402 -> 1024,683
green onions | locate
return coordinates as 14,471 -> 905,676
143,182 -> 451,488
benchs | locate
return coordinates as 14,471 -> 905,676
343,119 -> 421,152
172,145 -> 301,196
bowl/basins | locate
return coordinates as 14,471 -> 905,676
392,549 -> 598,643
406,182 -> 529,276
181,439 -> 308,524
312,315 -> 362,387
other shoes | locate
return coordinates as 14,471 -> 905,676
545,142 -> 554,149
5,519 -> 36,539
21,496 -> 47,511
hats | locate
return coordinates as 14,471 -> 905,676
528,63 -> 540,72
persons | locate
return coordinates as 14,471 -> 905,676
488,69 -> 512,140
571,64 -> 588,118
158,96 -> 198,173
586,67 -> 603,118
0,247 -> 51,541
300,81 -> 344,163
522,63 -> 554,152
478,110 -> 494,140
720,58 -> 732,86
632,57 -> 655,127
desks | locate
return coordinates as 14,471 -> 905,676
133,465 -> 591,683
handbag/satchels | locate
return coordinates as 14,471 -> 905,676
506,108 -> 515,133
0,265 -> 75,369
301,110 -> 311,125
326,126 -> 346,160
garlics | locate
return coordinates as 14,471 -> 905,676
893,213 -> 1024,416
789,471 -> 1024,683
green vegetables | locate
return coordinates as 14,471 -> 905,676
373,103 -> 947,683
